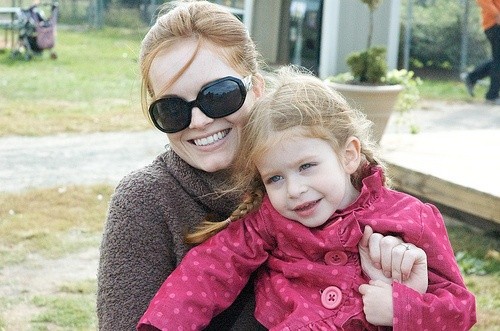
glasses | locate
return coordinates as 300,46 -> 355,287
148,75 -> 250,134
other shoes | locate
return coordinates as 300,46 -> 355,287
459,69 -> 474,97
478,93 -> 499,105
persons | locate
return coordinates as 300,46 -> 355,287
97,0 -> 428,331
132,63 -> 476,331
460,1 -> 500,103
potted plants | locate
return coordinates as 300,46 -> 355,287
324,1 -> 423,146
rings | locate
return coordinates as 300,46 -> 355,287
400,240 -> 411,251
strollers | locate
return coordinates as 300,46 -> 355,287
10,2 -> 59,61
